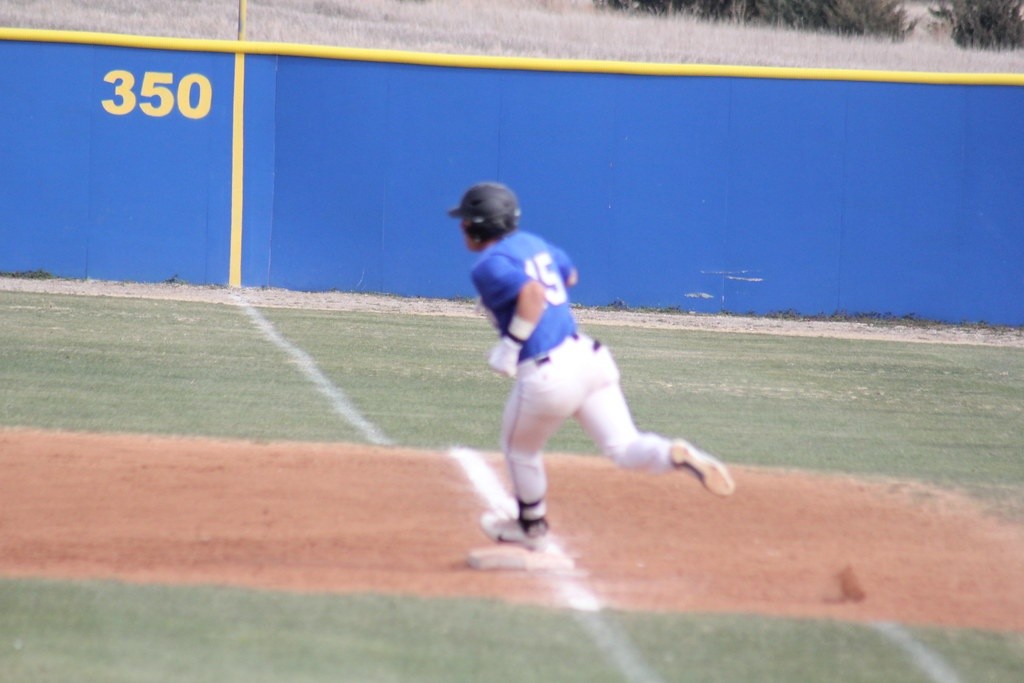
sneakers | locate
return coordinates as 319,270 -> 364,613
669,435 -> 737,497
477,507 -> 549,552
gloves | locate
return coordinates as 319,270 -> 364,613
487,334 -> 521,378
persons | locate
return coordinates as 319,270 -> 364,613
448,183 -> 736,552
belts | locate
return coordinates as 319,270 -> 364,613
536,332 -> 579,364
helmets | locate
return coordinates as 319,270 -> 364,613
449,183 -> 518,238
